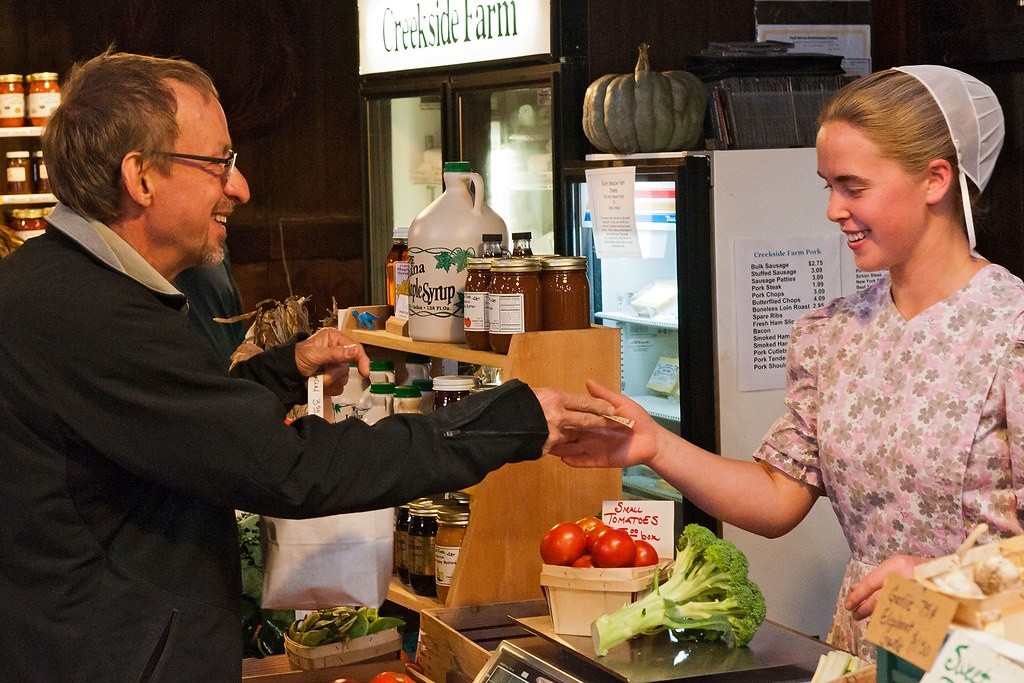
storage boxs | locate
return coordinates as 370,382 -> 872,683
413,594 -> 620,683
541,557 -> 674,635
283,628 -> 403,673
722,90 -> 836,147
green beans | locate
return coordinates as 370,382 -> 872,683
290,605 -> 407,648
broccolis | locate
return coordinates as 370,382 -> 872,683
592,522 -> 767,655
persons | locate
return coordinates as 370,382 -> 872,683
170,239 -> 244,370
0,39 -> 624,682
548,64 -> 1024,664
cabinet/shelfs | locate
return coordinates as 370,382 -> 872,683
337,303 -> 623,617
0,125 -> 60,205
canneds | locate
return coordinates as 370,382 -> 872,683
462,254 -> 590,355
393,491 -> 473,605
0,71 -> 62,247
432,375 -> 500,414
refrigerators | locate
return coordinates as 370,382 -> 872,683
555,146 -> 890,645
354,0 -> 923,305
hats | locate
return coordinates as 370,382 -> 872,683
892,65 -> 1006,204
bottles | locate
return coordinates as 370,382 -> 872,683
406,161 -> 509,343
330,354 -> 435,428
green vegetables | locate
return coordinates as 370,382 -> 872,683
238,510 -> 299,660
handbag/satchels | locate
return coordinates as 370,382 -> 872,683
260,376 -> 395,607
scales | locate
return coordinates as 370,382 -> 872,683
470,616 -> 839,683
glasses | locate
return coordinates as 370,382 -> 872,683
116,150 -> 237,175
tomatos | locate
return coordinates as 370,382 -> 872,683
329,671 -> 417,683
540,516 -> 659,568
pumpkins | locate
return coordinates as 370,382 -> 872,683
581,41 -> 709,153
